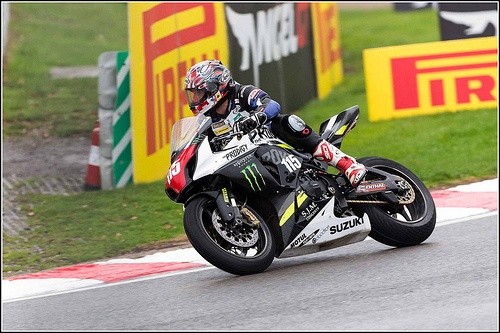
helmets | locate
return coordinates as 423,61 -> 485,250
184,59 -> 234,116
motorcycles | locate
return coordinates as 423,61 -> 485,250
163,103 -> 437,276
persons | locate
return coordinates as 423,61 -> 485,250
184,59 -> 367,188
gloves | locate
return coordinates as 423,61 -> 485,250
231,111 -> 268,137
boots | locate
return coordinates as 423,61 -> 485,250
313,138 -> 367,187
